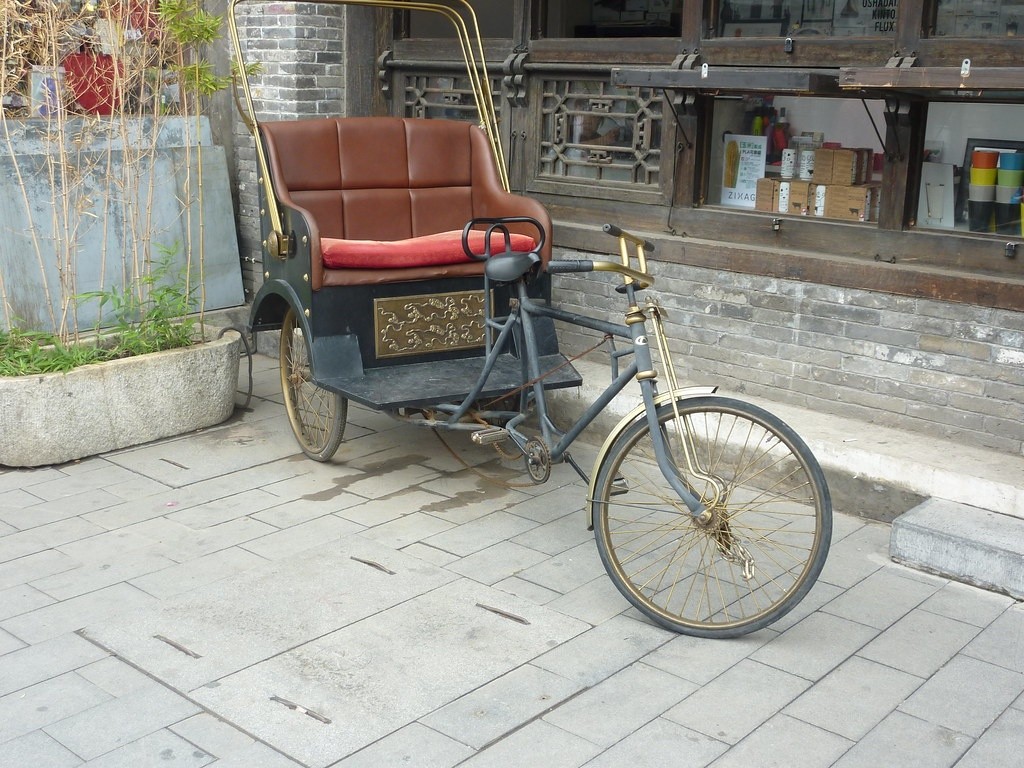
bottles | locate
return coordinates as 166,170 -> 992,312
751,107 -> 788,158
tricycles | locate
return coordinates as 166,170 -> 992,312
227,0 -> 833,640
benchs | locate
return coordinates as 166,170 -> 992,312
256,117 -> 553,292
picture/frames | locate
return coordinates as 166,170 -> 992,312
720,18 -> 789,38
954,137 -> 1024,224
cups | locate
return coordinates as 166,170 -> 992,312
863,151 -> 869,182
780,149 -> 797,179
801,151 -> 815,181
852,152 -> 858,184
873,188 -> 881,221
969,150 -> 1024,237
779,182 -> 790,212
815,184 -> 826,218
865,188 -> 872,220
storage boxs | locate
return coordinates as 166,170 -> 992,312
755,146 -> 881,220
934,0 -> 1024,35
591,0 -> 682,27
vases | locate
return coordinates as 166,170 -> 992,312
968,150 -> 1024,233
